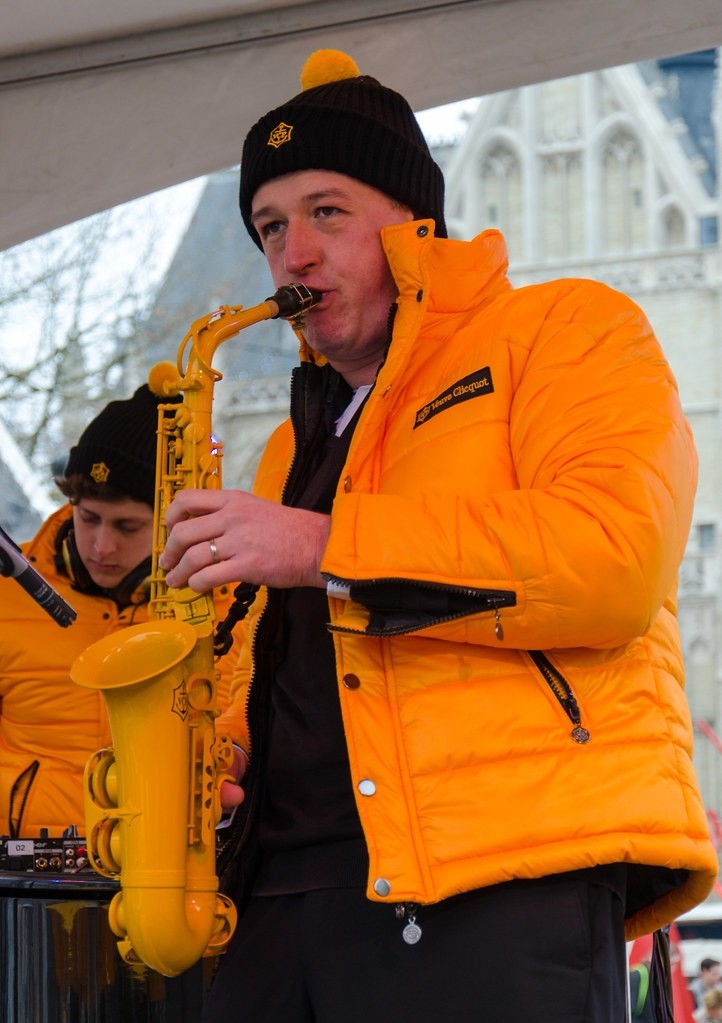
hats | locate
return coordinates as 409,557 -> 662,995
236,45 -> 448,255
61,359 -> 187,506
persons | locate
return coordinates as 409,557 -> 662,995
159,74 -> 717,1023
0,384 -> 244,995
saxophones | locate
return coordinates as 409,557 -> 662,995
66,276 -> 330,975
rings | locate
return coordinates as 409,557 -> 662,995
210,539 -> 219,561
